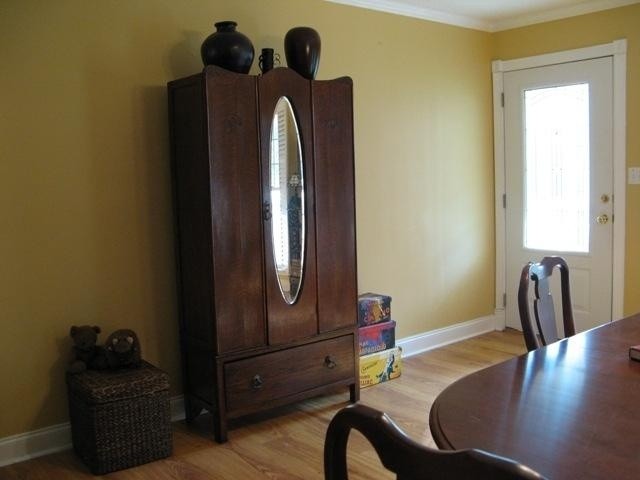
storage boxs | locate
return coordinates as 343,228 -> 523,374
359,346 -> 403,389
358,292 -> 392,328
360,319 -> 397,357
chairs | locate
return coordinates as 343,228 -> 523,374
517,256 -> 575,352
322,404 -> 549,480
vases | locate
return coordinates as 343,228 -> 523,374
200,21 -> 255,75
285,26 -> 321,79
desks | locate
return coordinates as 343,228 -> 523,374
430,307 -> 640,480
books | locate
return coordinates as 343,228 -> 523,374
629,343 -> 640,362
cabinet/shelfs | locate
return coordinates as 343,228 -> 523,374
168,64 -> 360,442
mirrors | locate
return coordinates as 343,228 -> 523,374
268,94 -> 309,305
